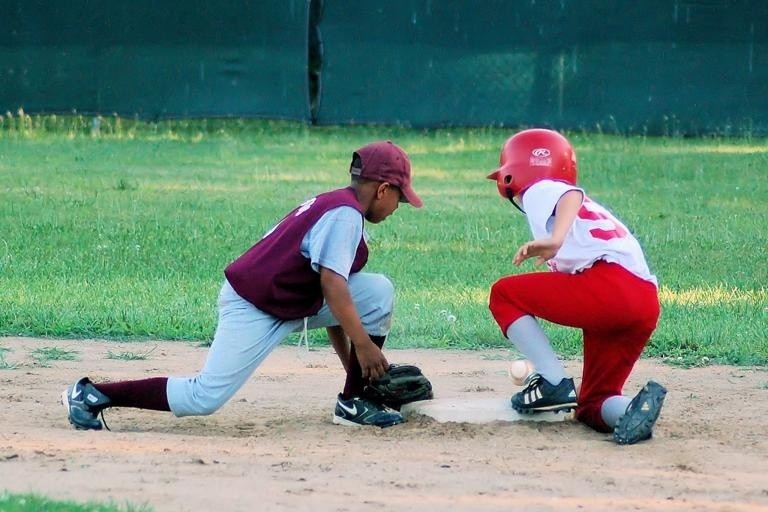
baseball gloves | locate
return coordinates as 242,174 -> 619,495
359,363 -> 433,411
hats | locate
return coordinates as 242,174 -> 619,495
349,140 -> 422,208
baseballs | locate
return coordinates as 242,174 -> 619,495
509,359 -> 530,385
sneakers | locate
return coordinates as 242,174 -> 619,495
614,380 -> 667,445
60,376 -> 111,431
510,373 -> 579,414
333,392 -> 405,428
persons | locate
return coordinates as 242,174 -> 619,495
61,140 -> 423,432
486,129 -> 668,446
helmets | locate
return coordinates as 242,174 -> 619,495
485,129 -> 577,199
